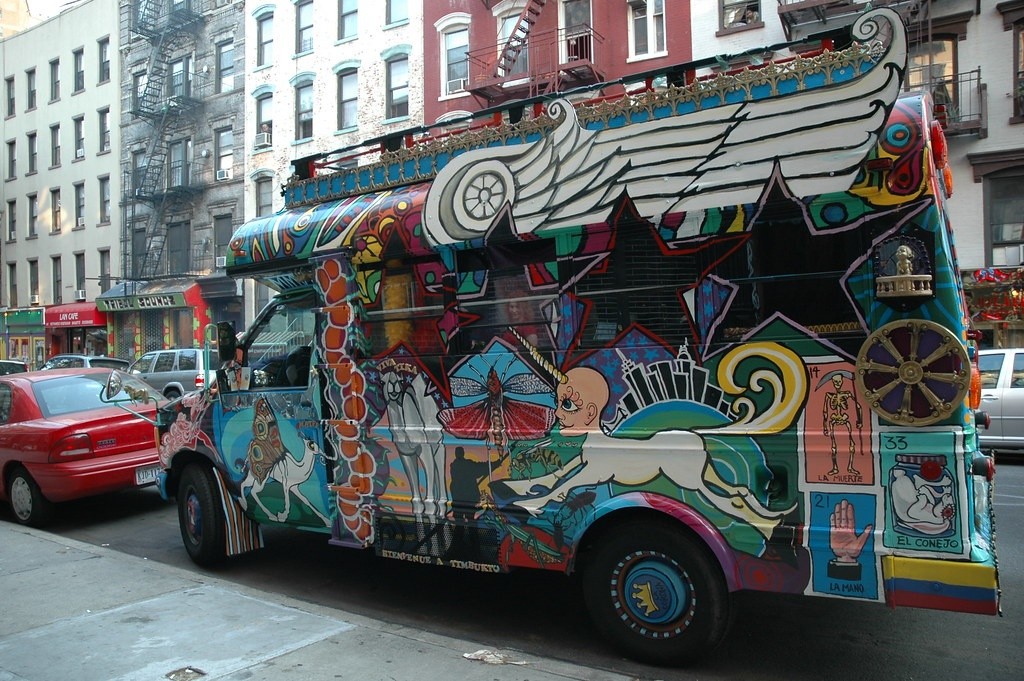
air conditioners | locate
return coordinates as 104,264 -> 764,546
256,133 -> 270,148
30,295 -> 39,304
217,170 -> 228,180
75,290 -> 86,301
216,257 -> 226,268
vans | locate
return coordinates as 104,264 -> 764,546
123,348 -> 219,402
0,359 -> 29,377
40,352 -> 130,375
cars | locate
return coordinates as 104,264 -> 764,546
976,347 -> 1024,461
0,368 -> 171,529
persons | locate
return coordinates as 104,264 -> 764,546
262,124 -> 271,134
746,10 -> 759,24
499,290 -> 537,347
895,245 -> 916,292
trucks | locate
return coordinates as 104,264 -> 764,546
99,7 -> 1004,671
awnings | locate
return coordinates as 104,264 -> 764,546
45,302 -> 107,328
